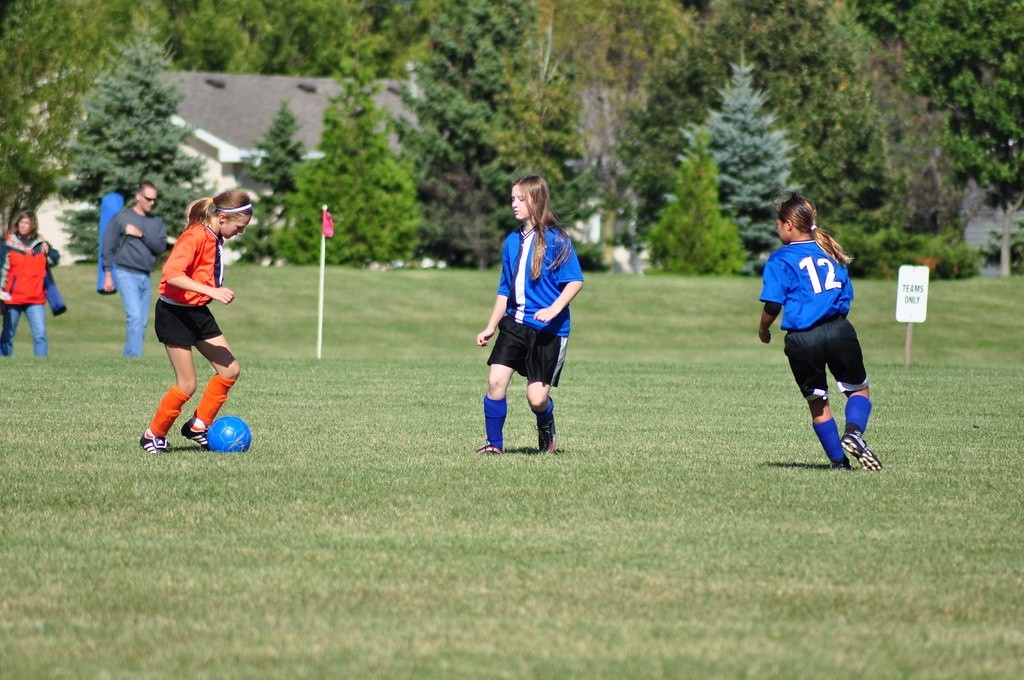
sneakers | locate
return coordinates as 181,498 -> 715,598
141,432 -> 171,453
831,457 -> 851,471
841,432 -> 882,471
181,422 -> 209,451
534,412 -> 556,453
477,438 -> 502,456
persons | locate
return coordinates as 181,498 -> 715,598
474,175 -> 586,455
759,196 -> 884,471
140,190 -> 253,454
0,211 -> 59,358
102,182 -> 167,356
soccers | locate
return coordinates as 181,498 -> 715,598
203,416 -> 250,451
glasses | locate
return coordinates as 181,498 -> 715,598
139,191 -> 156,202
